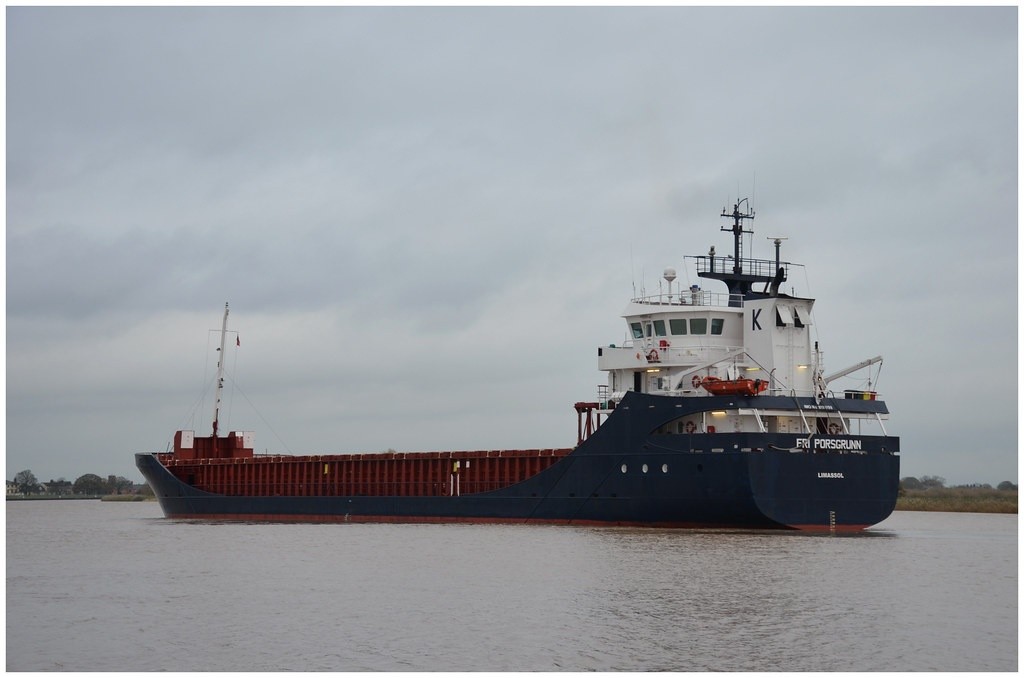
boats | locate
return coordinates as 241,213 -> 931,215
133,198 -> 900,530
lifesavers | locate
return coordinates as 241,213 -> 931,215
829,422 -> 839,434
686,420 -> 695,433
691,375 -> 701,389
648,350 -> 660,360
